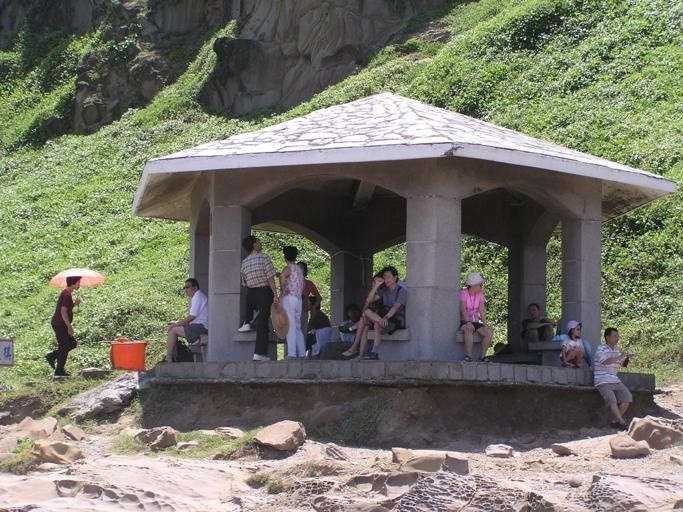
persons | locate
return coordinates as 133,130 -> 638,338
45,276 -> 82,379
157,278 -> 208,363
365,266 -> 406,359
520,303 -> 554,343
305,296 -> 331,355
278,246 -> 306,360
341,273 -> 384,360
459,273 -> 492,362
238,235 -> 280,360
560,320 -> 586,369
593,327 -> 632,429
296,262 -> 321,351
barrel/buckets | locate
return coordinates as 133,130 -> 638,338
109,337 -> 148,372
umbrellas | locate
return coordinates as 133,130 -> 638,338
48,267 -> 106,314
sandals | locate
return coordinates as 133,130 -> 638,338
464,355 -> 473,362
479,357 -> 493,364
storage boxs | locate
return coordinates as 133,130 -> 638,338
111,340 -> 148,372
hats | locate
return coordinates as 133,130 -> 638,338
566,319 -> 583,334
270,301 -> 289,341
464,273 -> 486,287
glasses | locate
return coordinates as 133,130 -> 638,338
183,285 -> 191,289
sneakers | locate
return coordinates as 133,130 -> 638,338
360,351 -> 379,361
238,322 -> 252,332
339,350 -> 360,359
252,353 -> 271,362
